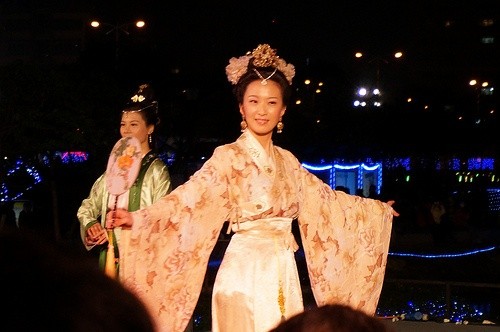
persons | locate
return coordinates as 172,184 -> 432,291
429,201 -> 452,247
76,84 -> 173,280
105,45 -> 399,332
